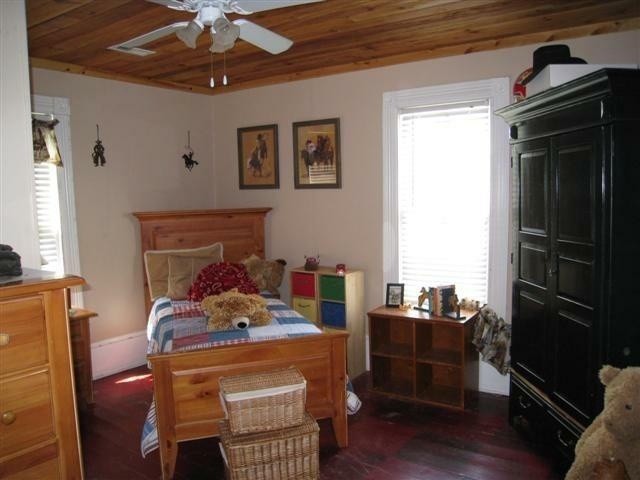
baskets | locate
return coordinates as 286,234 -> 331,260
219,407 -> 321,480
217,364 -> 307,437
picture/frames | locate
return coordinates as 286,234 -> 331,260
290,117 -> 343,190
384,282 -> 406,309
236,122 -> 282,190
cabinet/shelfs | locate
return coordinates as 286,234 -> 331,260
363,302 -> 481,410
66,305 -> 101,419
1,267 -> 92,480
287,263 -> 368,384
492,65 -> 640,478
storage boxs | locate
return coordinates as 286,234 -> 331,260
521,60 -> 639,100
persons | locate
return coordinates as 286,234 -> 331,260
251,134 -> 268,176
305,134 -> 331,165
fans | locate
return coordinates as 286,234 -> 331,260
107,0 -> 327,59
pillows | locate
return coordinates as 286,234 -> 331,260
238,255 -> 285,292
165,255 -> 222,301
142,240 -> 224,303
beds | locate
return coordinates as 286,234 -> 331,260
127,202 -> 354,479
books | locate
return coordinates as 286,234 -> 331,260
427,284 -> 455,317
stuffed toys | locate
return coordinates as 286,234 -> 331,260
243,258 -> 287,297
201,287 -> 272,332
563,365 -> 640,480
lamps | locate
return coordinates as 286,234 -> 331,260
175,0 -> 243,57
